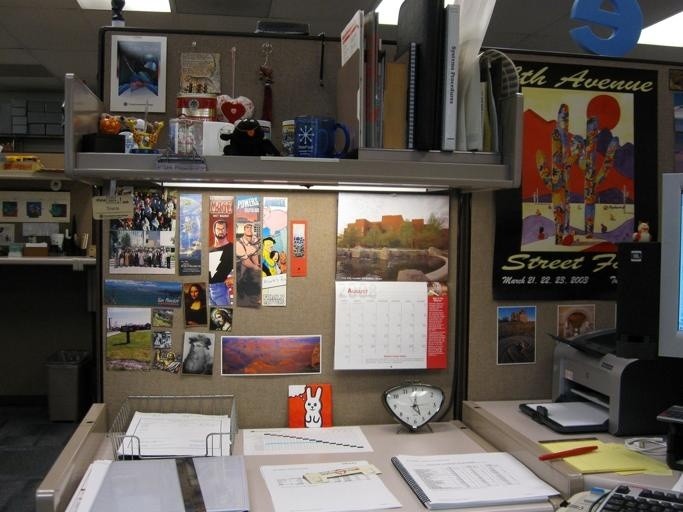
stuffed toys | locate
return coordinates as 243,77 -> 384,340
220,113 -> 285,158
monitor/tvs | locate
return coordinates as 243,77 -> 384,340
658,173 -> 683,358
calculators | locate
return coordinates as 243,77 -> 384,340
656,404 -> 683,423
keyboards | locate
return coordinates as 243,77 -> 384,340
594,484 -> 683,512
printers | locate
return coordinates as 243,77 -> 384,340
519,327 -> 683,441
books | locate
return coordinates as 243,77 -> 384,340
60,450 -> 258,511
331,1 -> 504,158
538,436 -> 648,475
389,446 -> 562,509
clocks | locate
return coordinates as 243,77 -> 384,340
384,380 -> 445,434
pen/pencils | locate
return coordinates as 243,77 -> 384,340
539,445 -> 597,461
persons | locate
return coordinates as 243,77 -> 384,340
236,223 -> 261,286
209,216 -> 234,307
107,188 -> 177,270
269,250 -> 281,274
182,333 -> 214,375
184,283 -> 207,326
210,308 -> 232,332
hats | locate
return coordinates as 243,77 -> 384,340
189,335 -> 212,350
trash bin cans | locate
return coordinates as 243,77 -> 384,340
40,344 -> 94,422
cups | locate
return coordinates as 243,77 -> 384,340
282,116 -> 351,159
51,234 -> 64,251
